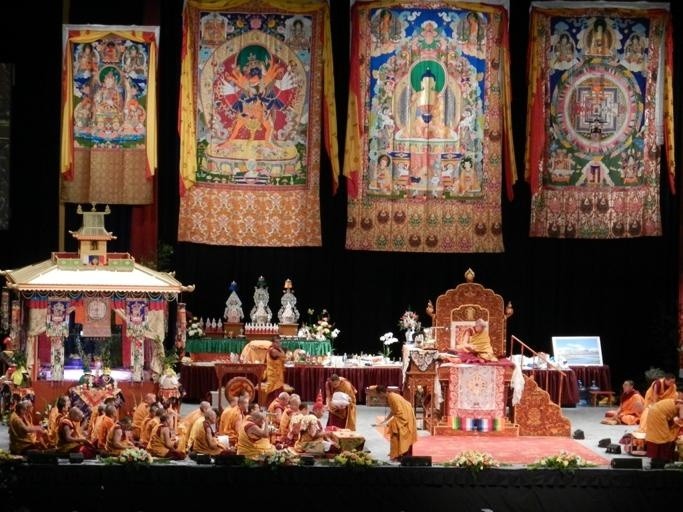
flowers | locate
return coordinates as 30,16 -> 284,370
521,451 -> 592,470
186,316 -> 203,340
398,311 -> 421,332
440,448 -> 502,472
307,308 -> 342,339
256,446 -> 310,466
379,332 -> 399,358
101,447 -> 154,465
329,446 -> 378,467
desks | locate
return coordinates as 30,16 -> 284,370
329,429 -> 366,451
179,359 -> 609,406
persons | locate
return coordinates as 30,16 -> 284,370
600,371 -> 683,469
73,38 -> 146,138
458,320 -> 500,364
370,9 -> 486,196
255,335 -> 285,392
199,11 -> 309,153
547,17 -> 648,184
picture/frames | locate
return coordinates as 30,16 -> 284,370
552,336 -> 604,367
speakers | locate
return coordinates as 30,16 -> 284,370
401,455 -> 431,467
611,458 -> 642,469
215,455 -> 245,465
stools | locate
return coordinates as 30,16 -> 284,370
588,390 -> 614,406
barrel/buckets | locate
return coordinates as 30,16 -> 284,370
210,390 -> 227,409
590,379 -> 600,406
578,379 -> 587,409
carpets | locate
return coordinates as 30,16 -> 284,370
411,431 -> 607,465
